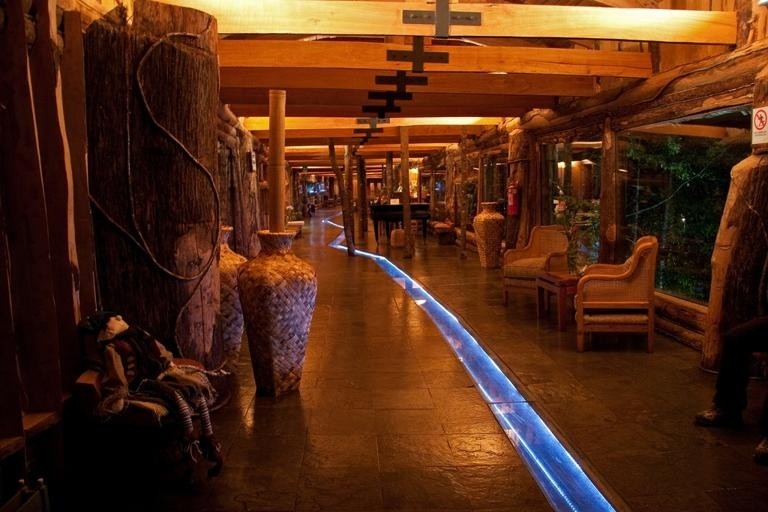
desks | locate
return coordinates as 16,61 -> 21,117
286,221 -> 305,238
536,271 -> 578,330
59,357 -> 206,497
370,204 -> 431,242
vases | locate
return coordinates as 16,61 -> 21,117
473,202 -> 505,269
238,229 -> 316,396
220,226 -> 248,360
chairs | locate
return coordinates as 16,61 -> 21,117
502,225 -> 569,311
574,236 -> 658,353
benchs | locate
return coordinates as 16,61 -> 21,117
429,220 -> 455,246
